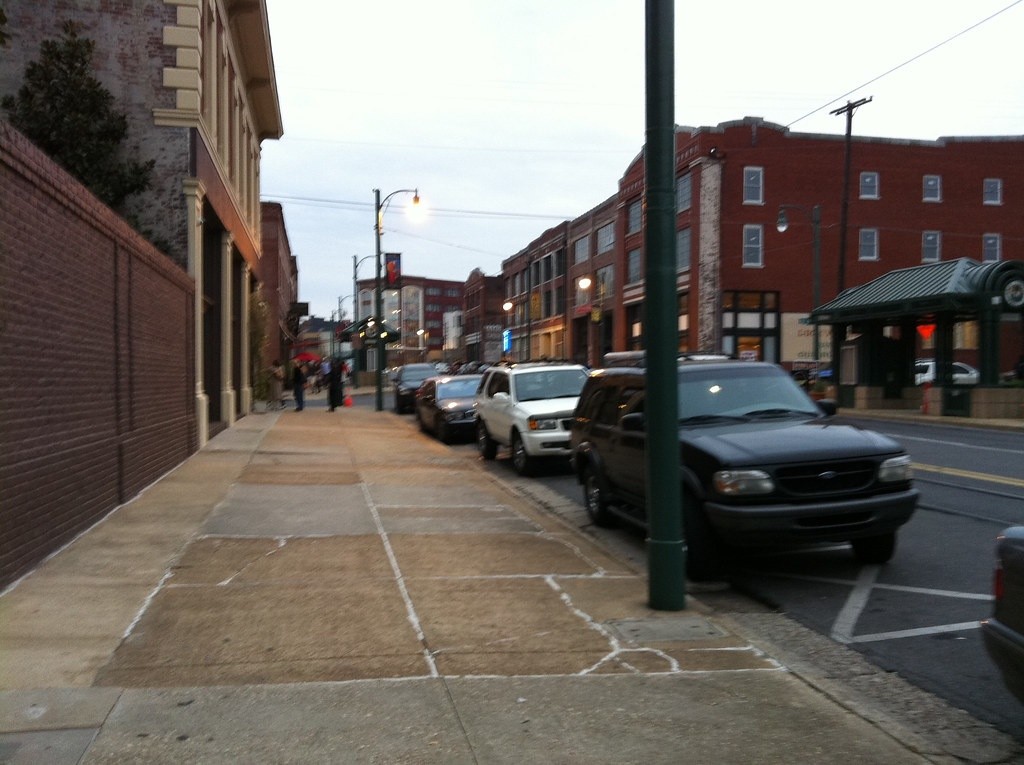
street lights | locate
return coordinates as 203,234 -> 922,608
373,187 -> 421,410
332,295 -> 358,356
502,289 -> 521,363
417,324 -> 447,364
579,272 -> 605,352
777,204 -> 820,363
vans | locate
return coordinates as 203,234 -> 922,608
602,351 -> 645,368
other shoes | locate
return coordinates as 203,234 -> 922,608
327,408 -> 334,412
296,407 -> 303,412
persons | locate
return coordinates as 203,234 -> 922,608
290,358 -> 351,411
271,360 -> 283,409
446,359 -> 509,375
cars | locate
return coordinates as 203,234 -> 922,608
678,354 -> 747,395
909,358 -> 981,388
435,363 -> 450,374
393,362 -> 438,413
415,376 -> 481,440
450,361 -> 493,375
983,521 -> 1024,707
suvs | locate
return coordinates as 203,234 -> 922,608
569,349 -> 919,576
474,363 -> 591,474
